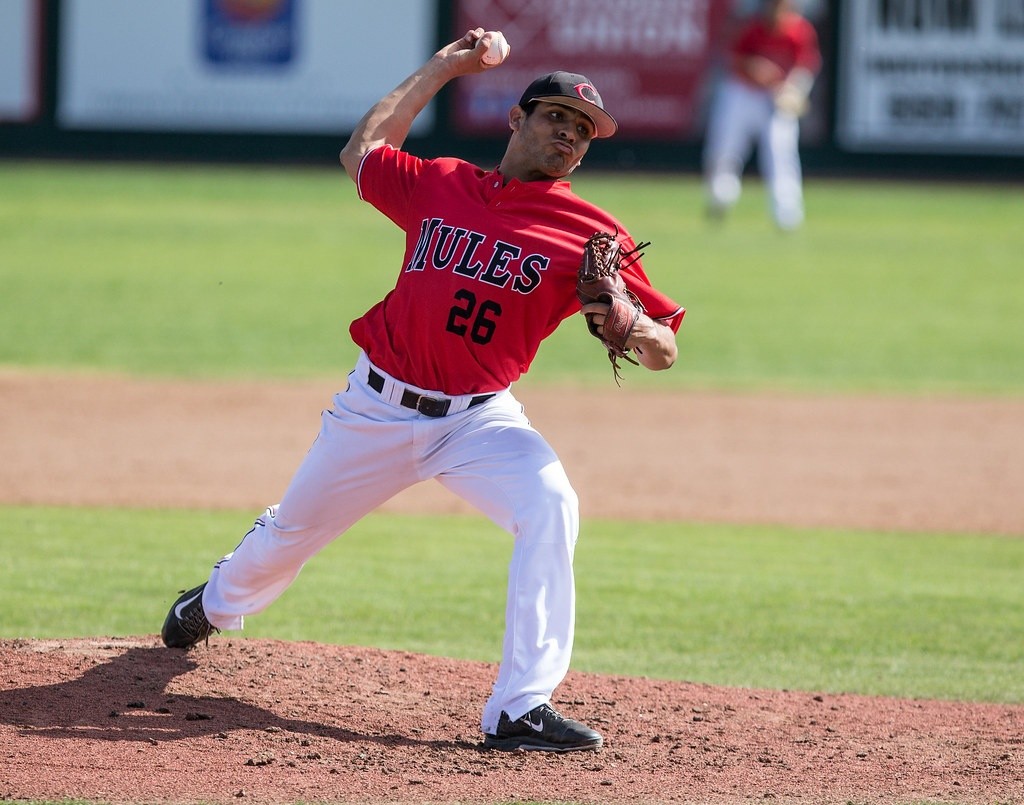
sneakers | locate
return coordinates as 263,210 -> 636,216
485,702 -> 603,752
161,581 -> 222,651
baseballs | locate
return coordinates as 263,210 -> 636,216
475,31 -> 509,66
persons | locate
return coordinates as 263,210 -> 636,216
160,28 -> 686,751
700,0 -> 822,228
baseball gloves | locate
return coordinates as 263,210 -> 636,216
573,230 -> 642,361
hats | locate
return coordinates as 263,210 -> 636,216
519,71 -> 619,140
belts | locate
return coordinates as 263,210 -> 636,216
368,367 -> 496,417
